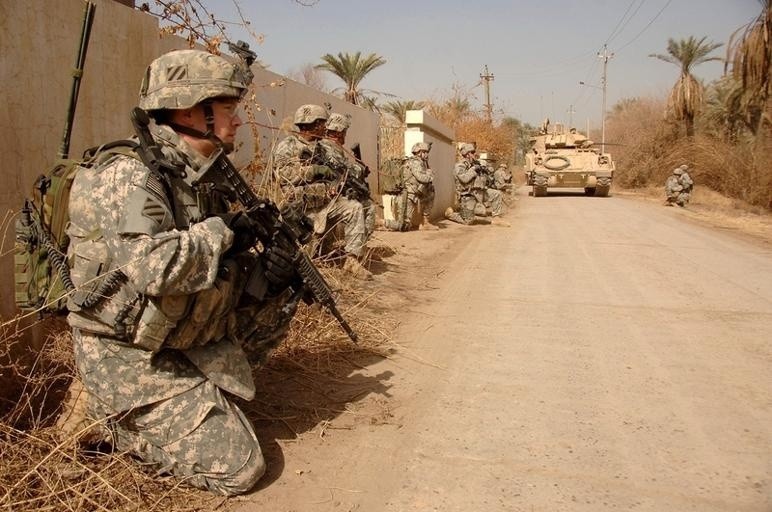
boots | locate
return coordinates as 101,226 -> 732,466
374,215 -> 385,228
419,214 -> 440,231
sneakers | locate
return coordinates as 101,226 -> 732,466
343,254 -> 372,281
491,216 -> 511,228
445,207 -> 453,218
54,382 -> 94,425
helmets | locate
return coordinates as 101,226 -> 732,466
138,50 -> 247,110
326,113 -> 350,132
500,164 -> 507,168
460,144 -> 475,155
673,164 -> 689,174
293,104 -> 327,124
411,141 -> 431,155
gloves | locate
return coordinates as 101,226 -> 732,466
218,211 -> 258,255
311,164 -> 336,181
472,164 -> 481,171
264,248 -> 297,287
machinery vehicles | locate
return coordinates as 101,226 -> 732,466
524,130 -> 617,198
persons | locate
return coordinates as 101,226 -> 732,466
374,142 -> 439,232
52,48 -> 304,498
269,104 -> 374,283
662,164 -> 694,208
444,143 -> 513,226
318,110 -> 375,268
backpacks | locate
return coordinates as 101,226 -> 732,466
380,159 -> 414,194
14,140 -> 173,316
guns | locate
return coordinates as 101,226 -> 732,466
470,158 -> 497,189
299,148 -> 383,209
190,148 -> 358,345
